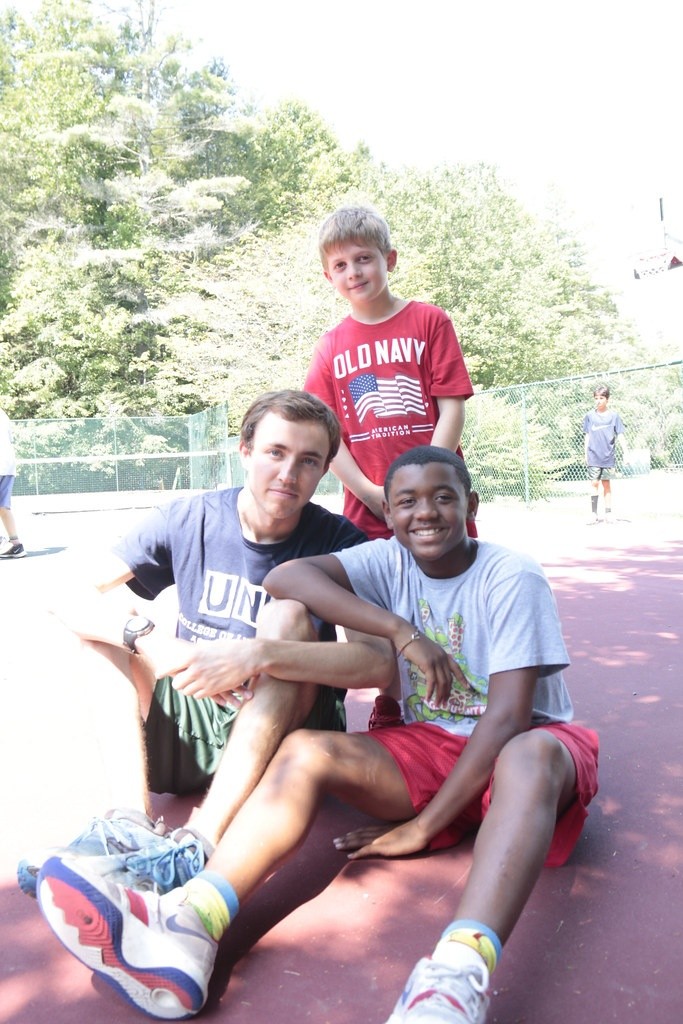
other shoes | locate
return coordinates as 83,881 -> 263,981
585,512 -> 614,525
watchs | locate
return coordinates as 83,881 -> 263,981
123,614 -> 156,656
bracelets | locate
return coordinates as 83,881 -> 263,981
397,625 -> 421,657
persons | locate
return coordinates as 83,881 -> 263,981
581,387 -> 625,523
34,445 -> 598,1022
305,206 -> 481,540
0,410 -> 28,558
17,390 -> 368,899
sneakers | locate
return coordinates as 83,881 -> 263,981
375,958 -> 491,1024
18,810 -> 215,899
35,855 -> 240,1021
0,541 -> 27,559
